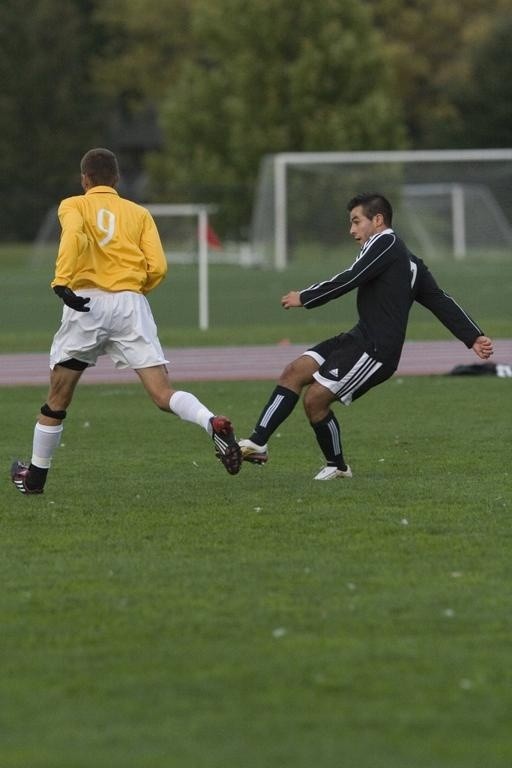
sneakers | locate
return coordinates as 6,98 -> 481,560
11,458 -> 44,497
313,464 -> 352,480
215,438 -> 268,466
208,416 -> 242,475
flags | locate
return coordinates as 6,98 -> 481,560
199,220 -> 222,250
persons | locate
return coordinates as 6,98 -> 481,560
11,146 -> 243,497
241,190 -> 496,484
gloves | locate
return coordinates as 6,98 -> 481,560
53,285 -> 91,312
